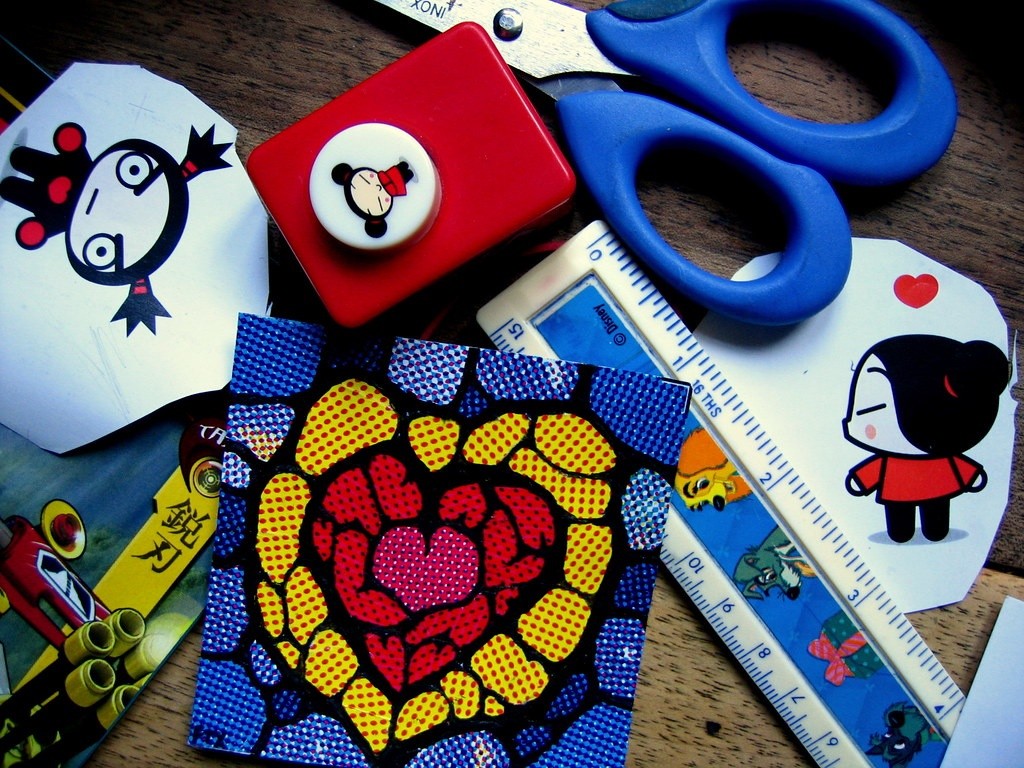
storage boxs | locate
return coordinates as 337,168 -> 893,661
244,21 -> 575,328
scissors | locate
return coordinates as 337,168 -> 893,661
377,2 -> 956,329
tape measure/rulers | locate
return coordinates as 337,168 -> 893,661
475,221 -> 967,768
